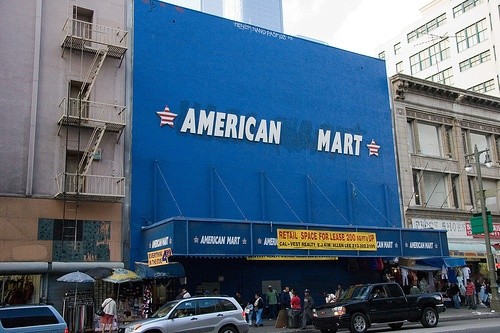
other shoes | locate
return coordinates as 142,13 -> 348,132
259,324 -> 263,326
254,324 -> 258,327
248,325 -> 252,327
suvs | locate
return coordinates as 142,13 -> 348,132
123,295 -> 249,333
0,304 -> 68,333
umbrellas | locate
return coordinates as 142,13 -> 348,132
56,270 -> 96,305
85,267 -> 115,303
101,269 -> 140,306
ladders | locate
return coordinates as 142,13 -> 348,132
60,82 -> 82,251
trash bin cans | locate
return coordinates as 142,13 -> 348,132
287,309 -> 300,328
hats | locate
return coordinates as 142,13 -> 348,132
305,289 -> 310,292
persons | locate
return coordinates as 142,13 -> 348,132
210,289 -> 220,296
265,284 -> 281,321
441,282 -> 462,310
323,291 -> 337,303
181,288 -> 191,298
280,286 -> 315,329
335,283 -> 345,302
235,292 -> 243,305
464,278 -> 491,310
410,283 -> 420,295
246,301 -> 254,326
100,293 -> 118,333
252,293 -> 264,327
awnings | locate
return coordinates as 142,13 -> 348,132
400,265 -> 441,272
134,262 -> 185,280
423,256 -> 466,269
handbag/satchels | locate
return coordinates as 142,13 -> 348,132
96,307 -> 105,316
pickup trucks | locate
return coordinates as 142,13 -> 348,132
311,281 -> 447,333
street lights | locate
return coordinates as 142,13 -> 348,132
463,143 -> 500,312
